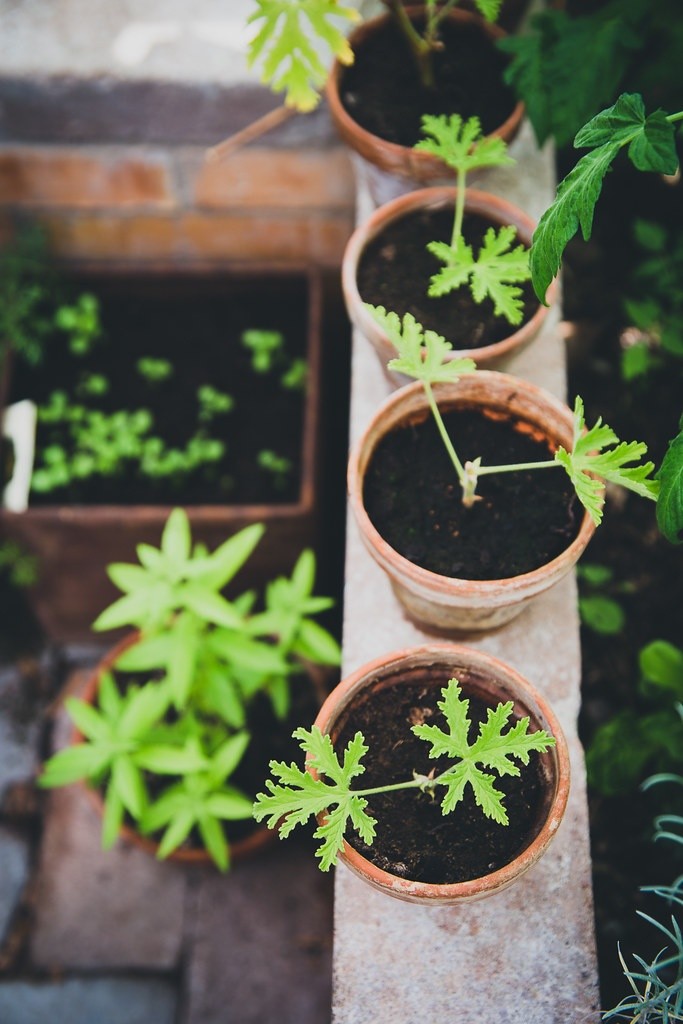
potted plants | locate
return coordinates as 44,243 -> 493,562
242,0 -> 527,208
2,212 -> 322,646
39,503 -> 342,874
350,295 -> 665,638
248,637 -> 572,905
338,110 -> 554,391
